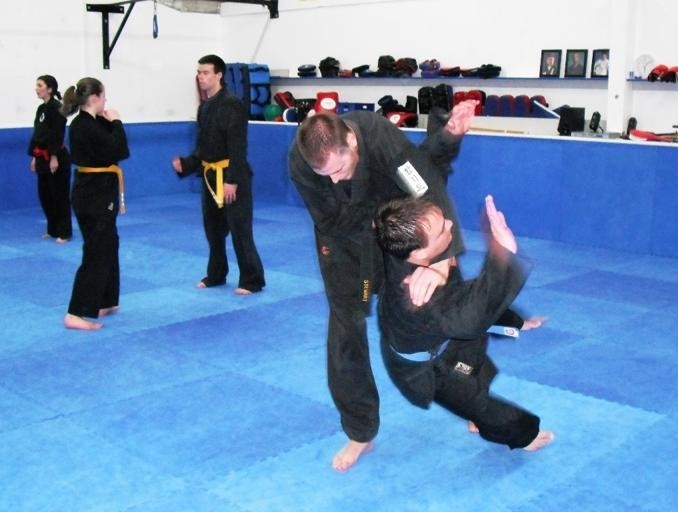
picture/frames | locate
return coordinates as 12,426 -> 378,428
540,49 -> 610,78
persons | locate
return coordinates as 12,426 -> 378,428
566,53 -> 584,75
542,52 -> 559,77
61,77 -> 130,332
375,99 -> 553,452
171,55 -> 265,294
288,110 -> 482,475
593,52 -> 609,77
26,75 -> 76,243
